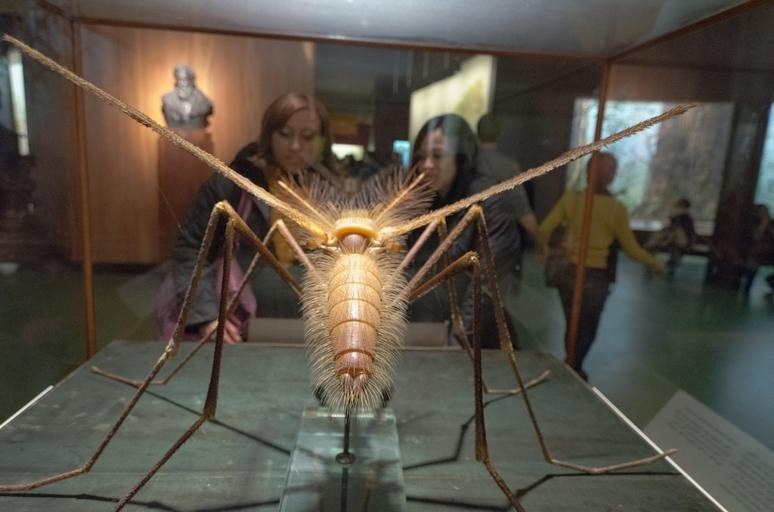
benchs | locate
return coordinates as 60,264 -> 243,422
644,236 -> 712,258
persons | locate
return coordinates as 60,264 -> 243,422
473,113 -> 541,240
533,151 -> 668,382
343,151 -> 400,179
643,197 -> 694,275
162,66 -> 213,129
731,204 -> 774,293
152,90 -> 338,344
402,113 -> 522,349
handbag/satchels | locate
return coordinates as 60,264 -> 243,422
156,257 -> 255,346
